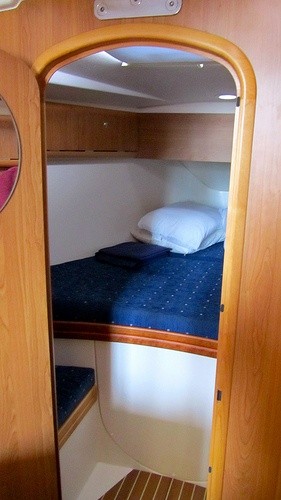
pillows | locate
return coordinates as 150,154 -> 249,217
131,201 -> 228,255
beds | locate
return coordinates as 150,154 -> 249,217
48,239 -> 228,485
